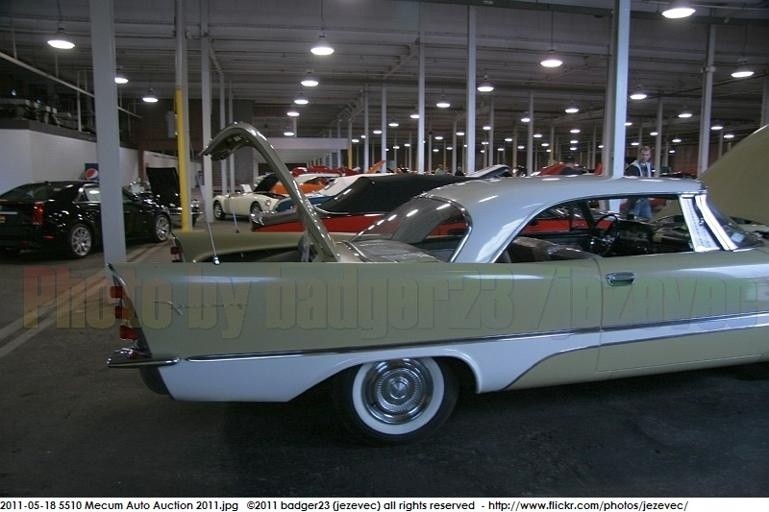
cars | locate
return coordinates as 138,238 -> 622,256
0,181 -> 172,257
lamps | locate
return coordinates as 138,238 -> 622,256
352,0 -> 754,153
283,0 -> 337,137
46,0 -> 159,104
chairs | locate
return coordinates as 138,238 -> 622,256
508,234 -> 559,264
545,245 -> 603,262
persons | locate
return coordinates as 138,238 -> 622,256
435,163 -> 444,176
623,146 -> 654,219
455,166 -> 465,176
